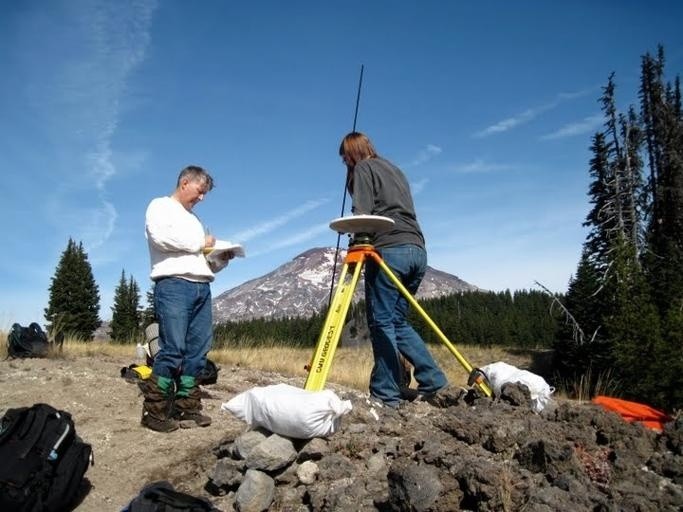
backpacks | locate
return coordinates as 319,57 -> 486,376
147,347 -> 218,383
5,322 -> 64,358
0,403 -> 95,512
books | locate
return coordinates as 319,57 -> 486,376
201,238 -> 232,255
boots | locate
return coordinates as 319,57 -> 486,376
141,379 -> 180,433
173,385 -> 212,428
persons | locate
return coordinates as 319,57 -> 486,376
338,130 -> 451,405
142,165 -> 237,434
